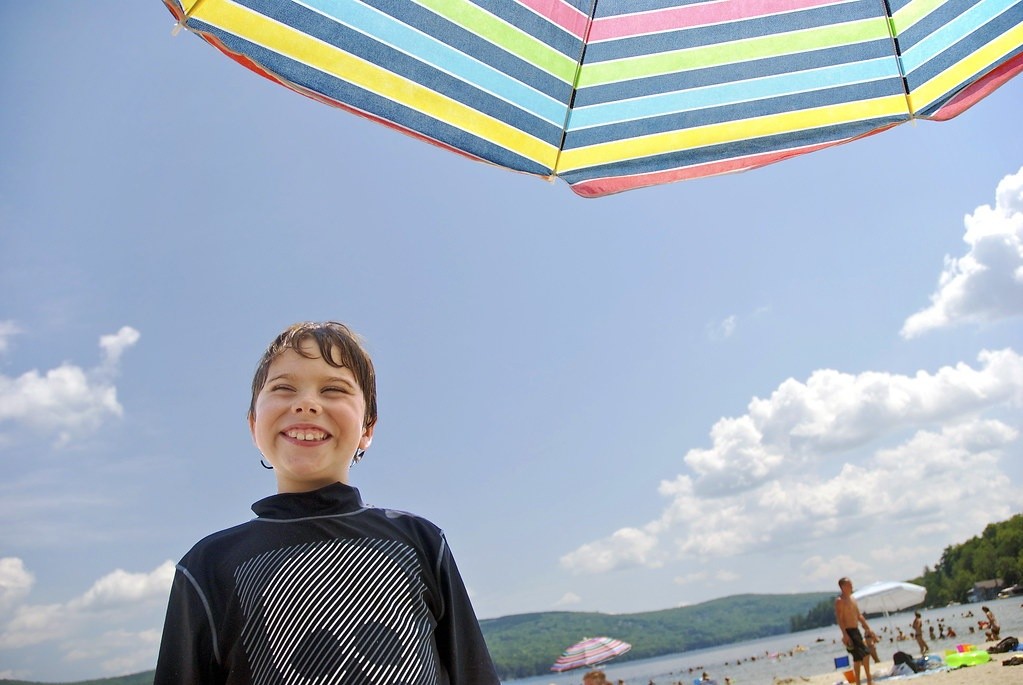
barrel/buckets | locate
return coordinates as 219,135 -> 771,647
957,644 -> 976,652
844,669 -> 854,683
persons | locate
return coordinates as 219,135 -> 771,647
862,623 -> 880,663
982,606 -> 1000,640
584,645 -> 801,685
155,323 -> 501,685
836,578 -> 873,685
913,610 -> 928,655
927,611 -> 993,642
877,624 -> 916,643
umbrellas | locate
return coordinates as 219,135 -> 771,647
851,580 -> 927,652
551,636 -> 632,674
160,0 -> 1023,202
